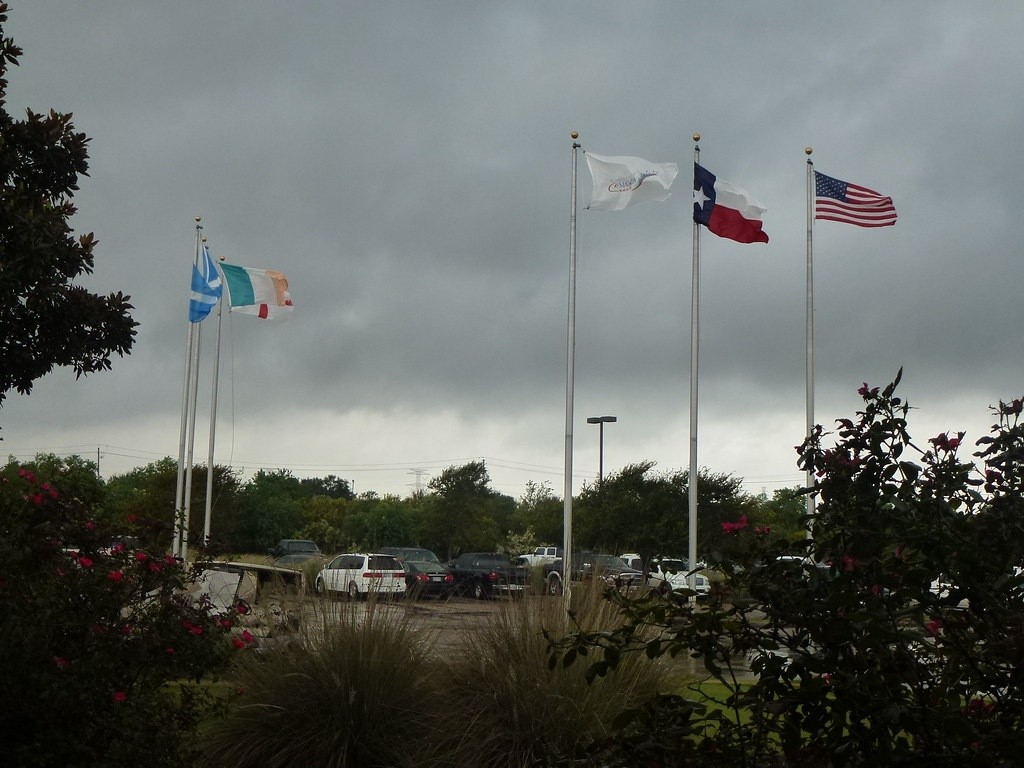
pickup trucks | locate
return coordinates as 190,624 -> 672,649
517,546 -> 591,569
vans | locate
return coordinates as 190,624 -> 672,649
190,561 -> 305,637
929,567 -> 1024,608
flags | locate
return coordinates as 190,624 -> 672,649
217,263 -> 294,319
189,248 -> 222,323
813,170 -> 898,227
584,152 -> 679,212
693,162 -> 769,244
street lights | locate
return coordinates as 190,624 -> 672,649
588,416 -> 617,489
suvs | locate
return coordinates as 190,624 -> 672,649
619,554 -> 711,598
268,538 -> 322,565
270,547 -> 452,600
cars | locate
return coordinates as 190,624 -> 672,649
541,553 -> 648,594
447,552 -> 537,600
